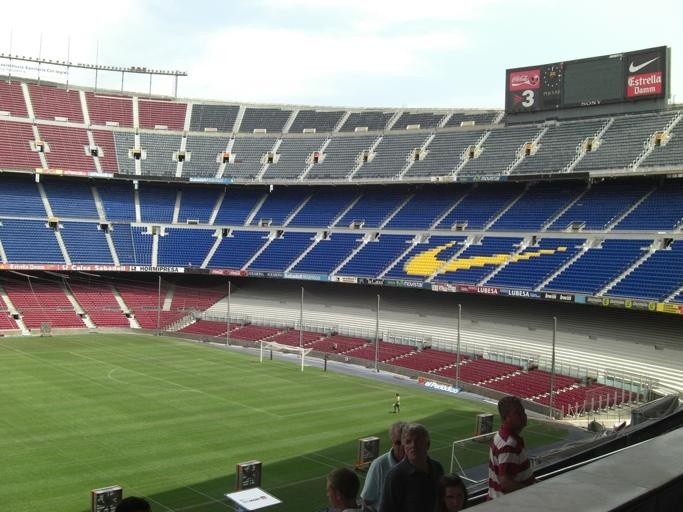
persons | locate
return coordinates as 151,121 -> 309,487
376,422 -> 446,512
431,471 -> 468,512
324,468 -> 370,512
113,495 -> 154,512
485,396 -> 536,502
359,421 -> 412,512
391,391 -> 400,413
